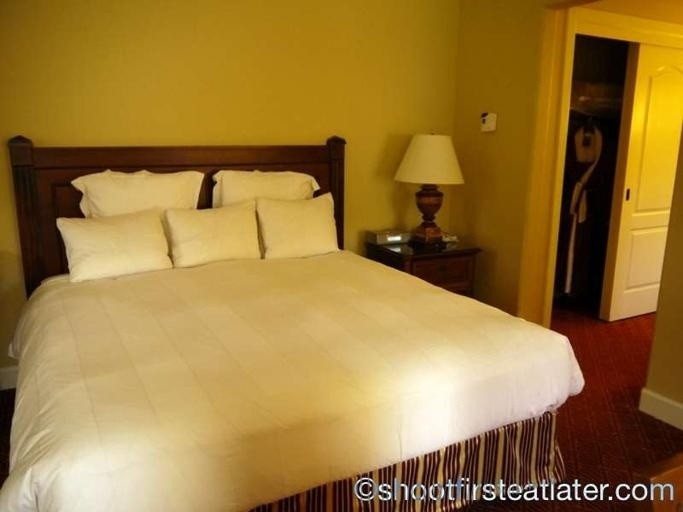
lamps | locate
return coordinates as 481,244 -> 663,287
392,135 -> 464,248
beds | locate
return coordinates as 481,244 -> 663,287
0,136 -> 585,512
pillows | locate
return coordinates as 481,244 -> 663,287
212,169 -> 320,207
166,201 -> 262,267
70,169 -> 205,218
56,212 -> 173,283
257,193 -> 339,260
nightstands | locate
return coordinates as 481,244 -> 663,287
365,241 -> 481,297
